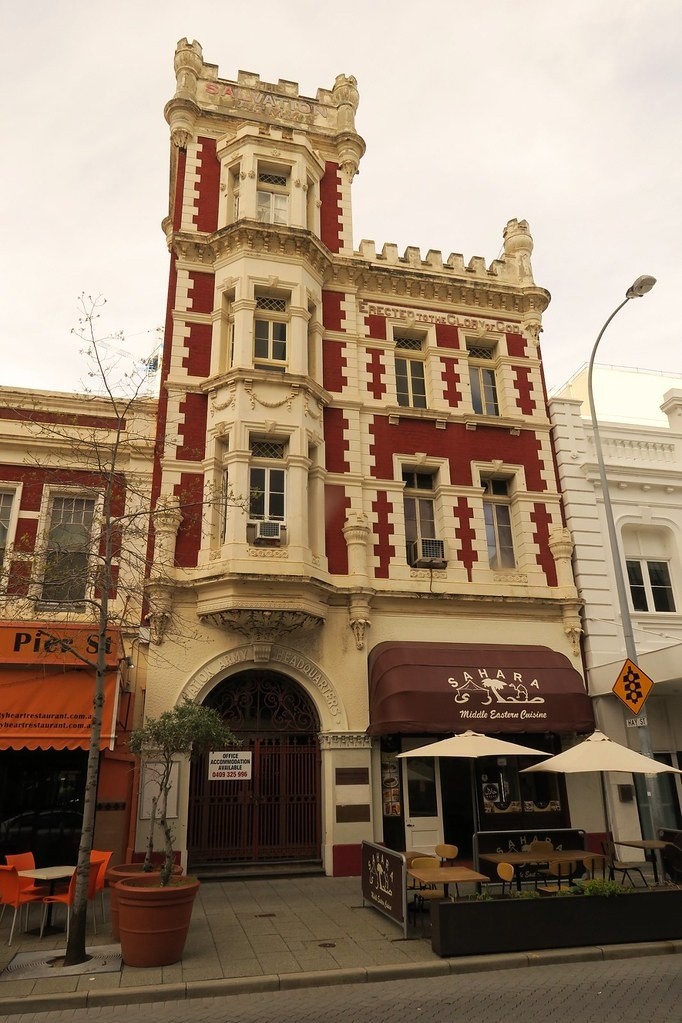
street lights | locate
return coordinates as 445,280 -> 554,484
587,274 -> 662,884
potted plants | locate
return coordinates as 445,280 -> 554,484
106,692 -> 246,968
430,877 -> 682,956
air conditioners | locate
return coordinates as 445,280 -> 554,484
410,537 -> 448,567
255,520 -> 281,541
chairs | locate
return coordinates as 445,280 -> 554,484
531,838 -> 648,897
497,862 -> 513,895
0,850 -> 115,944
406,844 -> 459,926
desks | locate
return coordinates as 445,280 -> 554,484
399,851 -> 435,890
541,849 -> 609,887
616,840 -> 671,885
406,867 -> 491,939
16,865 -> 78,937
478,852 -> 550,892
